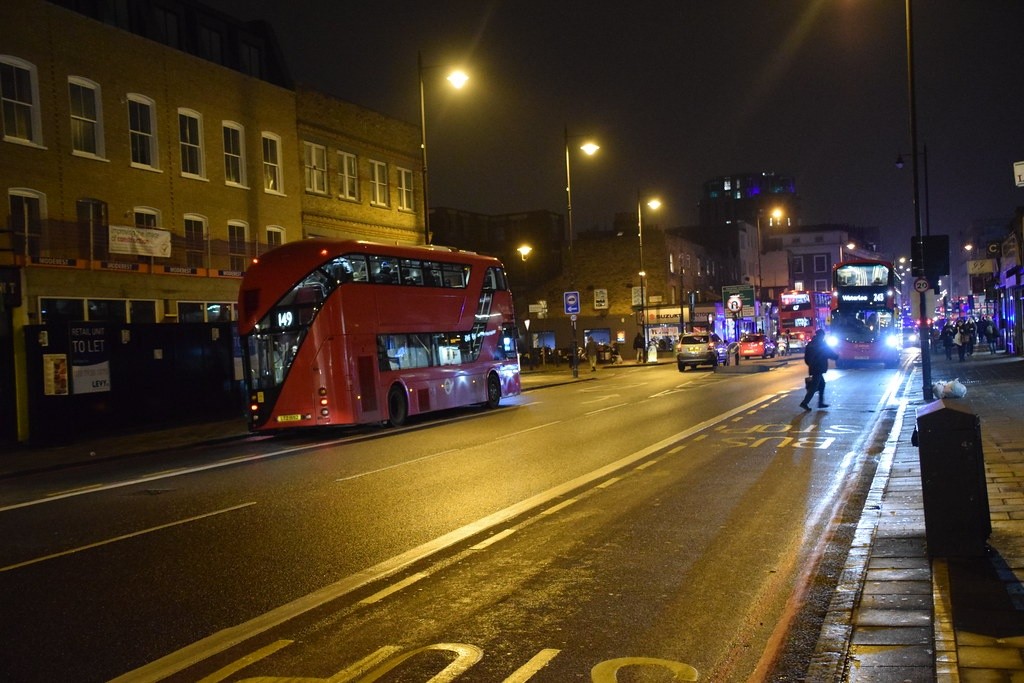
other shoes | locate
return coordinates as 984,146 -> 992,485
818,402 -> 829,408
800,402 -> 812,411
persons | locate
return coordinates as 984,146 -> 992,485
305,257 -> 354,295
633,332 -> 643,362
261,345 -> 283,382
370,258 -> 451,287
610,346 -> 623,363
938,316 -> 1000,362
585,337 -> 598,370
799,329 -> 839,411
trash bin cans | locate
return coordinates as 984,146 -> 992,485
912,408 -> 992,556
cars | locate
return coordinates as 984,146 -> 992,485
738,333 -> 775,359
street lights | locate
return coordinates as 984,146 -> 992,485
636,185 -> 663,363
894,140 -> 939,356
839,235 -> 856,264
417,49 -> 473,246
516,243 -> 534,371
756,206 -> 784,333
564,120 -> 599,379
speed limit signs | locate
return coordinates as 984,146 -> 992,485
914,277 -> 929,293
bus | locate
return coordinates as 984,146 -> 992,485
828,258 -> 904,370
236,237 -> 523,440
777,289 -> 832,353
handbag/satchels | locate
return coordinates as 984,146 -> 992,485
987,326 -> 994,334
805,374 -> 819,392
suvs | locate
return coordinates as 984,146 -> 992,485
676,332 -> 730,372
